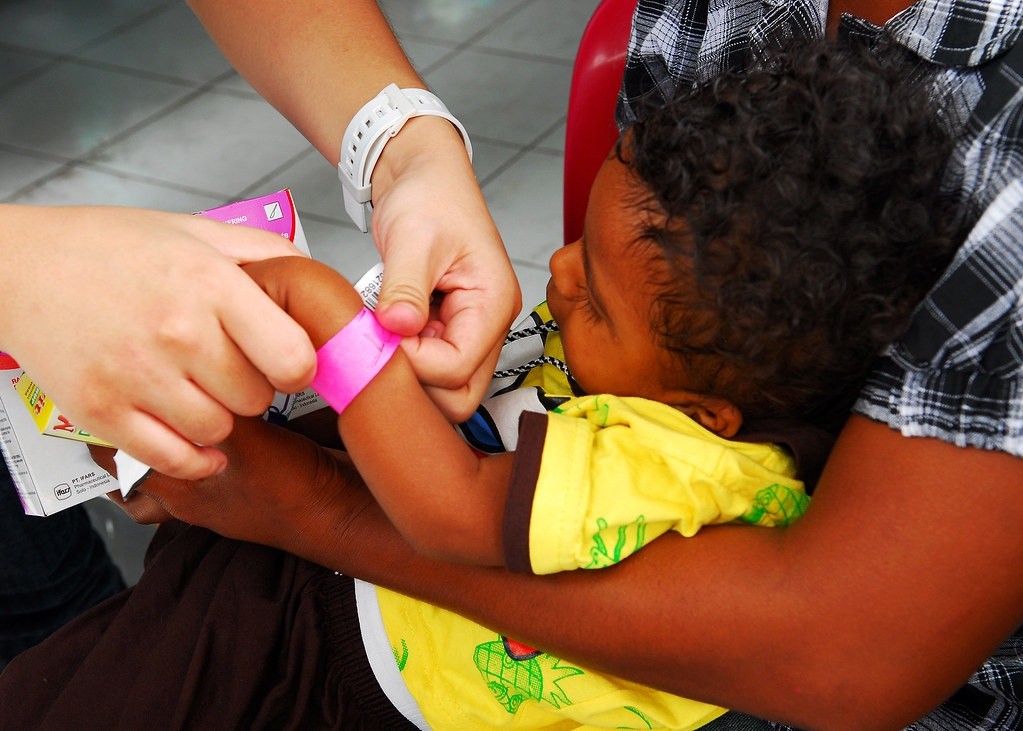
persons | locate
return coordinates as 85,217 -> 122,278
0,0 -> 1023,731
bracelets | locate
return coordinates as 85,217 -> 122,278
311,305 -> 402,414
337,83 -> 473,234
